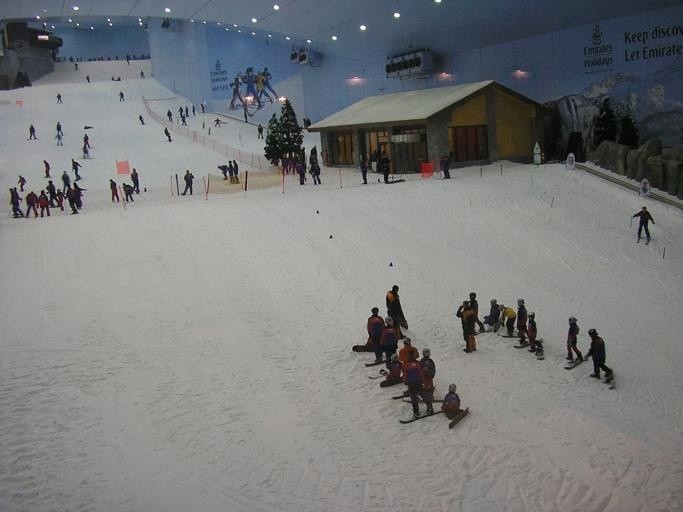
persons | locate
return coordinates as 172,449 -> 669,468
366,284 -> 460,421
164,104 -> 205,143
565,316 -> 583,361
528,312 -> 538,353
256,71 -> 274,104
56,93 -> 63,104
484,299 -> 500,331
282,145 -> 322,185
218,159 -> 240,184
633,207 -> 655,245
10,121 -> 92,217
516,299 -> 529,344
110,168 -> 140,202
215,117 -> 221,127
303,119 -> 308,129
584,328 -> 613,385
180,169 -> 194,195
138,114 -> 145,125
498,304 -> 517,337
120,91 -> 124,102
74,62 -> 79,71
440,152 -> 451,179
112,76 -> 121,82
306,118 -> 311,127
358,150 -> 390,185
140,70 -> 145,79
469,292 -> 484,333
126,56 -> 130,65
456,300 -> 483,354
258,124 -> 262,139
264,67 -> 279,99
230,70 -> 274,111
87,75 -> 90,83
